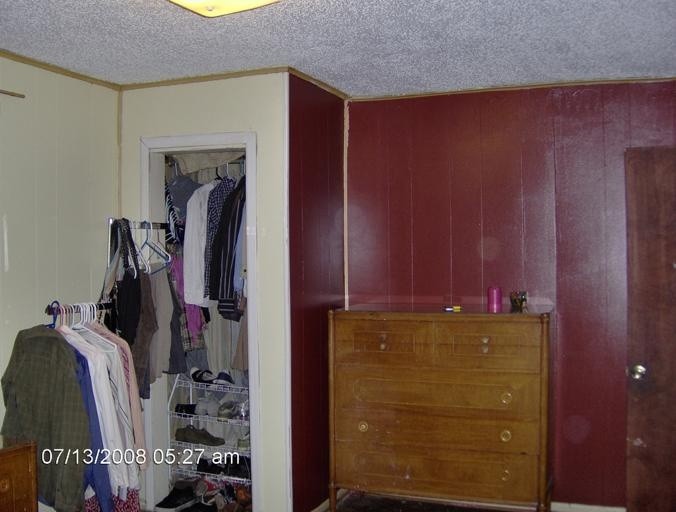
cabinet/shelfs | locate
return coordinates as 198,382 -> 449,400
168,373 -> 251,488
0,435 -> 37,512
327,302 -> 559,512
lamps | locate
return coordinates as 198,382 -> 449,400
168,0 -> 280,18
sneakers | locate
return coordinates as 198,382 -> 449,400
216,370 -> 238,386
217,401 -> 251,423
178,453 -> 224,474
153,480 -> 196,511
175,424 -> 204,442
184,428 -> 226,446
237,433 -> 250,451
190,367 -> 218,385
175,397 -> 222,418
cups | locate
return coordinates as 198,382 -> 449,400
487,287 -> 503,313
509,291 -> 528,313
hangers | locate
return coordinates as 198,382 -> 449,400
116,217 -> 172,280
44,300 -> 130,359
181,157 -> 230,187
